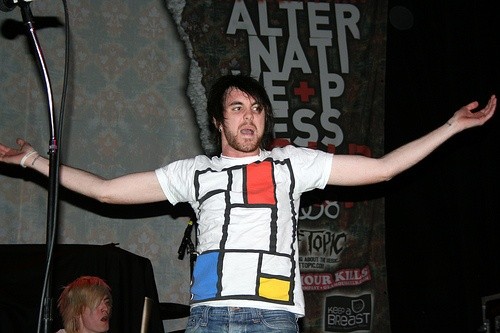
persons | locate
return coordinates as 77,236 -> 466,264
0,73 -> 497,333
54,276 -> 114,333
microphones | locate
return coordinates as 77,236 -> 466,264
0,0 -> 32,12
178,219 -> 194,254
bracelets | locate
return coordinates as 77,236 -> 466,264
21,149 -> 39,168
